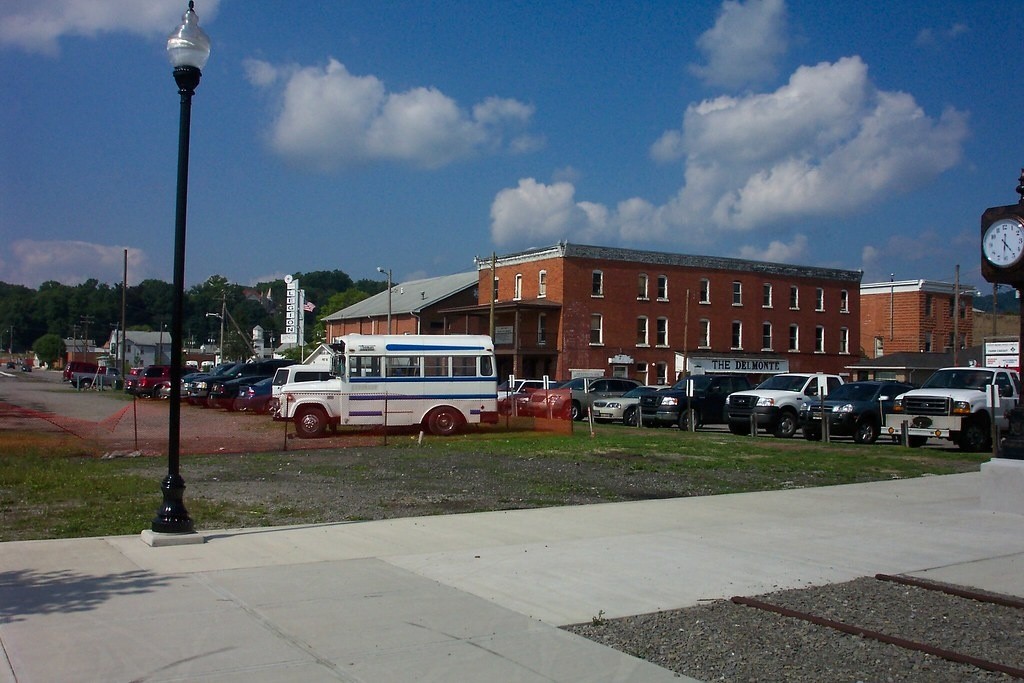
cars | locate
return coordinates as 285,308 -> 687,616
62,359 -> 300,413
7,362 -> 16,369
494,379 -> 557,417
234,377 -> 280,415
524,377 -> 643,421
21,364 -> 32,373
801,378 -> 928,446
591,384 -> 673,426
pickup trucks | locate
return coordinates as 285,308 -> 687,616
892,365 -> 1024,451
723,373 -> 845,438
638,374 -> 763,431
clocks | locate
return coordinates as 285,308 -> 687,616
981,205 -> 1024,285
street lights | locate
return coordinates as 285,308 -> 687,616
147,1 -> 200,535
472,255 -> 497,345
376,265 -> 392,336
205,312 -> 225,366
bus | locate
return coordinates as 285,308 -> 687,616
268,333 -> 499,440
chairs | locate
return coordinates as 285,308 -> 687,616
396,362 -> 489,376
